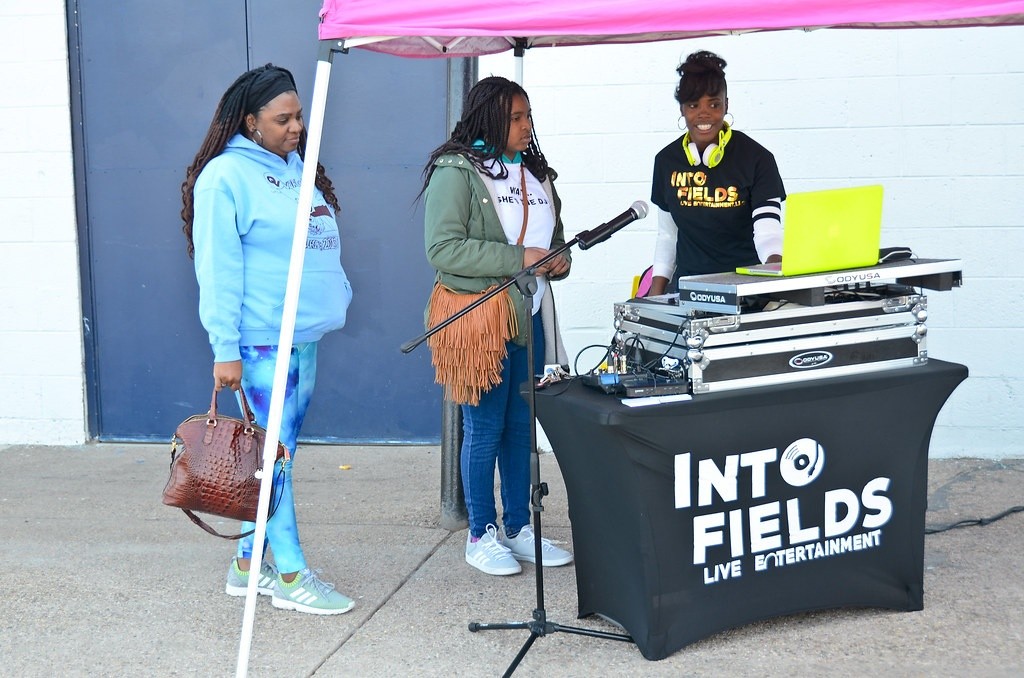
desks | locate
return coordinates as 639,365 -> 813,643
521,358 -> 970,661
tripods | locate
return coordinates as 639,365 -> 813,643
400,211 -> 640,678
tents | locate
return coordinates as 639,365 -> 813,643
236,0 -> 1024,678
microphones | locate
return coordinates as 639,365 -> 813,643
579,200 -> 649,250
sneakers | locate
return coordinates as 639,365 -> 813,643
498,523 -> 573,565
271,568 -> 354,615
463,523 -> 521,577
224,558 -> 279,597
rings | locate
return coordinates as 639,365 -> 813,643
222,383 -> 226,387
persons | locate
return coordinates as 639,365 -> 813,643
645,49 -> 786,297
411,76 -> 574,578
178,63 -> 357,614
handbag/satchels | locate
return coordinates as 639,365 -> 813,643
162,384 -> 290,541
429,283 -> 514,408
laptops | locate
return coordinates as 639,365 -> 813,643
734,183 -> 882,277
